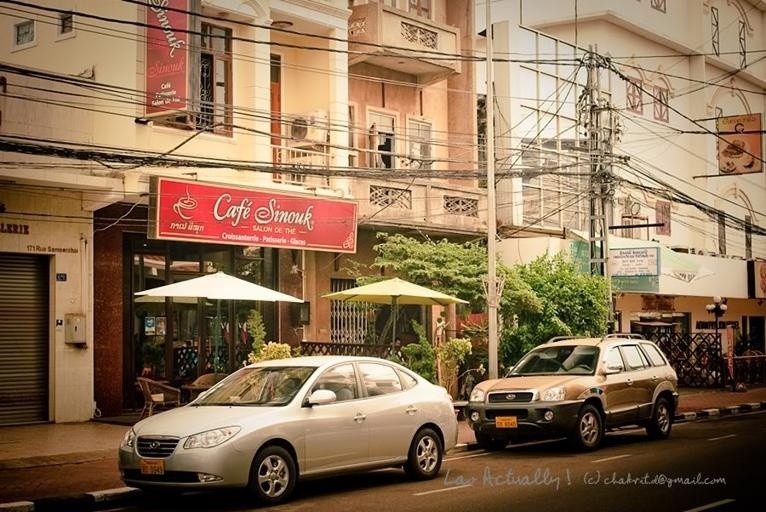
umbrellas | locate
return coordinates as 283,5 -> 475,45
322,276 -> 470,359
134,271 -> 306,386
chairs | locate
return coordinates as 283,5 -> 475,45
137,374 -> 181,419
192,373 -> 228,385
275,379 -> 297,401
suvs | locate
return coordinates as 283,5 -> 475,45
463,333 -> 679,452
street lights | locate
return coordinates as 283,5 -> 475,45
705,294 -> 728,392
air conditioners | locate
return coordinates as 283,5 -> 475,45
285,109 -> 327,186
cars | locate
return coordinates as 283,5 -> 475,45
118,355 -> 460,505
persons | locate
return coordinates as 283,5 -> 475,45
383,337 -> 406,360
277,379 -> 298,394
326,375 -> 353,400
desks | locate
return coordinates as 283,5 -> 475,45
181,383 -> 211,401
